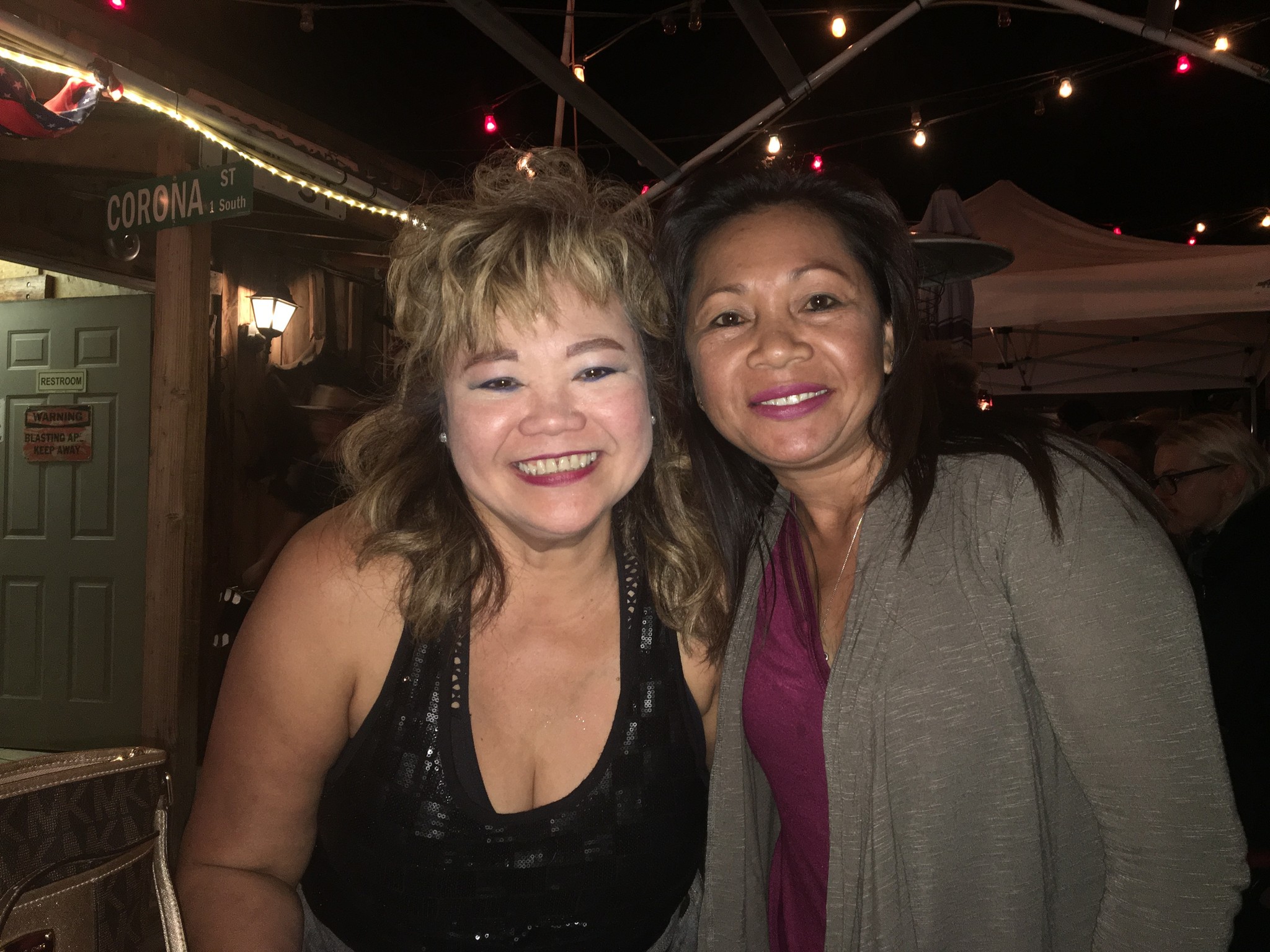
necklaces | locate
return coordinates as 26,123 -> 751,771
793,495 -> 868,663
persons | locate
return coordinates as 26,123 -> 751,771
183,205 -> 724,947
676,176 -> 1241,948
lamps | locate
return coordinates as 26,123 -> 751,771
241,280 -> 302,368
